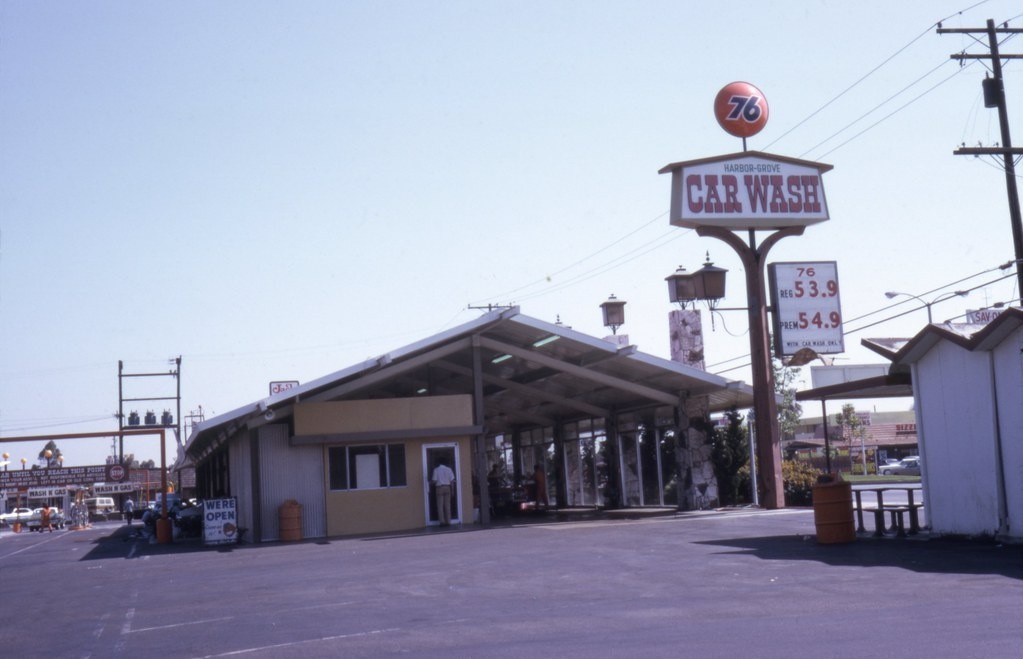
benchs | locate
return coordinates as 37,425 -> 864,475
854,502 -> 926,537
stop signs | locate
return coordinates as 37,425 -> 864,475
105,463 -> 129,482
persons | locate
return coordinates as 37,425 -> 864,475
124,495 -> 134,522
489,462 -> 505,504
431,456 -> 456,527
40,502 -> 53,533
528,464 -> 549,510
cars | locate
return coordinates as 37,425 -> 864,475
0,506 -> 65,532
879,455 -> 920,475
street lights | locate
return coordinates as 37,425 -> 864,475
20,458 -> 27,470
885,290 -> 969,323
2,452 -> 9,471
57,456 -> 64,467
44,450 -> 53,468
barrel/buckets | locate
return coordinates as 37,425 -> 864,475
813,481 -> 857,544
279,503 -> 302,541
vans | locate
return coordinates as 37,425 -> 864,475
85,497 -> 115,518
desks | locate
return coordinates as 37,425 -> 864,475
851,486 -> 923,537
